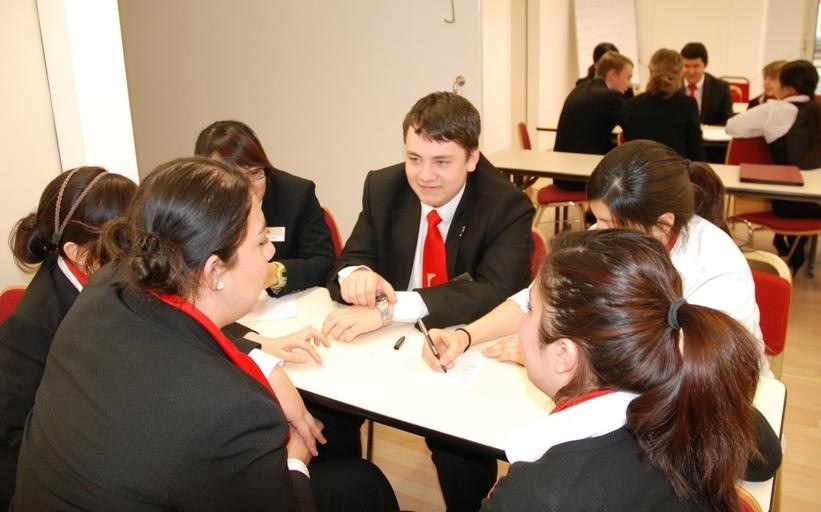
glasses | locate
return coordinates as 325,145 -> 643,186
241,165 -> 269,183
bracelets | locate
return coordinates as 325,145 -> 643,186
455,328 -> 471,351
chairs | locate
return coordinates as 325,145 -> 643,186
367,230 -> 545,463
320,208 -> 342,259
701,135 -> 820,375
517,122 -> 623,236
1,286 -> 28,322
721,77 -> 752,104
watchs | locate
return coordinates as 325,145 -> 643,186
13,156 -> 400,512
374,294 -> 393,326
270,261 -> 287,292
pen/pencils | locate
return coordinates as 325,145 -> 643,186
417,317 -> 448,374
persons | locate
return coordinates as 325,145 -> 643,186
194,121 -> 336,299
477,228 -> 781,512
307,93 -> 536,512
0,167 -> 140,512
423,139 -> 775,379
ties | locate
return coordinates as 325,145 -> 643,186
689,84 -> 699,97
419,209 -> 452,291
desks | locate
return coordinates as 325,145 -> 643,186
234,286 -> 787,512
486,149 -> 819,203
700,101 -> 750,143
